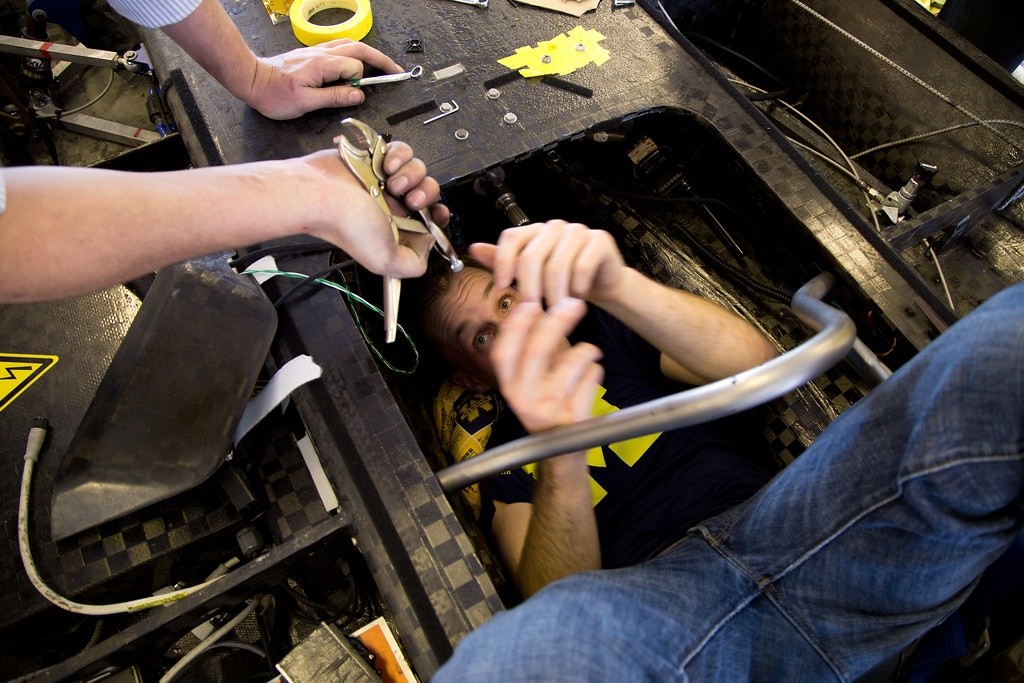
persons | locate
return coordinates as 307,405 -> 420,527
415,217 -> 1024,683
0,2 -> 453,309
